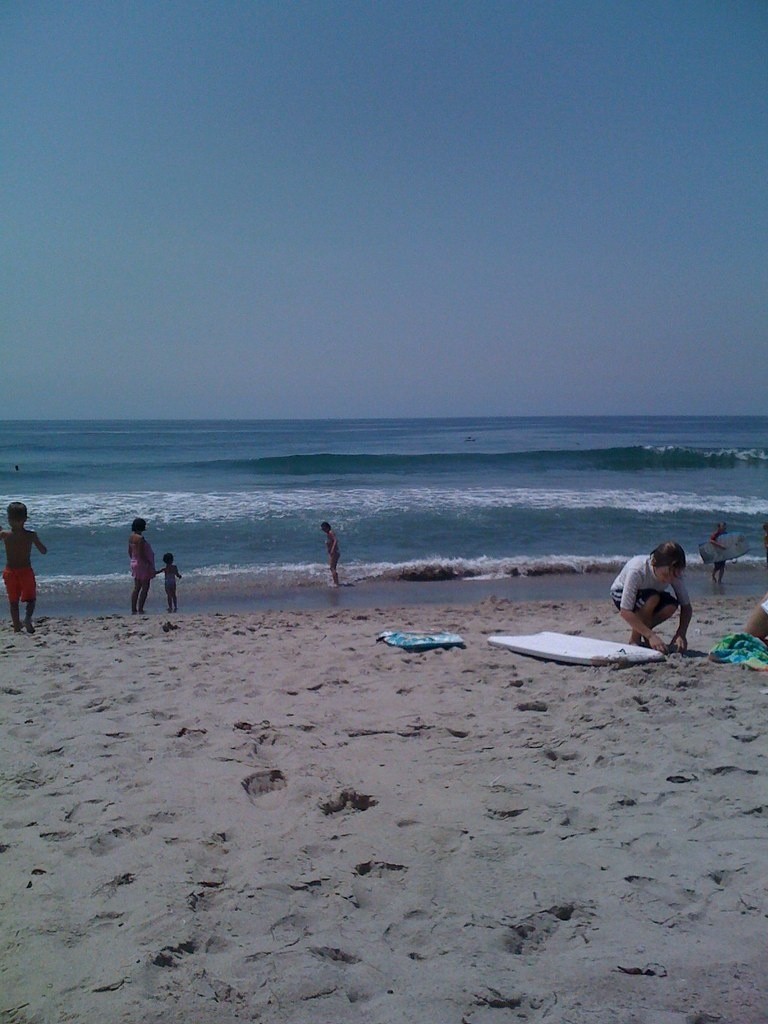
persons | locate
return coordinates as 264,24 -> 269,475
611,541 -> 691,652
763,524 -> 768,569
15,466 -> 18,471
746,591 -> 768,646
155,553 -> 181,611
321,522 -> 339,588
128,519 -> 153,612
712,521 -> 727,584
0,502 -> 46,632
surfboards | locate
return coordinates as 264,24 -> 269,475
487,630 -> 666,666
697,532 -> 751,564
